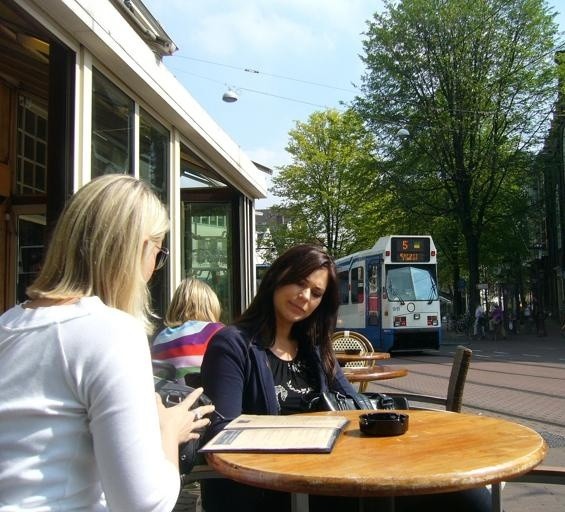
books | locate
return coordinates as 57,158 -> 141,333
195,411 -> 351,455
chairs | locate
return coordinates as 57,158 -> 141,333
329,330 -> 474,412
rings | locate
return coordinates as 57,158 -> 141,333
191,410 -> 201,420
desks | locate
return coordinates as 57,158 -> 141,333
204,405 -> 549,512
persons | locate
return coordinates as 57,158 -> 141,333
199,247 -> 497,511
153,277 -> 226,393
0,170 -> 215,512
460,298 -> 556,342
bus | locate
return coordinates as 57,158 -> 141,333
328,230 -> 444,356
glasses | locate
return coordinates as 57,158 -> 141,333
154,245 -> 171,271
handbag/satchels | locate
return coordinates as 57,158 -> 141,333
153,375 -> 215,476
302,392 -> 409,413
